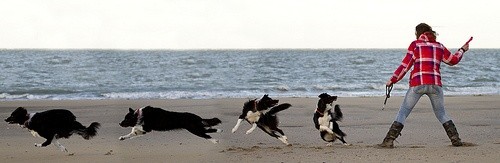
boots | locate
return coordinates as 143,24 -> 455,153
442,120 -> 465,147
379,121 -> 404,148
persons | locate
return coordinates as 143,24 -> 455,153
377,23 -> 470,148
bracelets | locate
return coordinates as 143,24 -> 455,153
460,47 -> 465,52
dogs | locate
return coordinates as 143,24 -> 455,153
119,105 -> 223,145
312,92 -> 350,147
232,94 -> 293,146
5,107 -> 102,155
